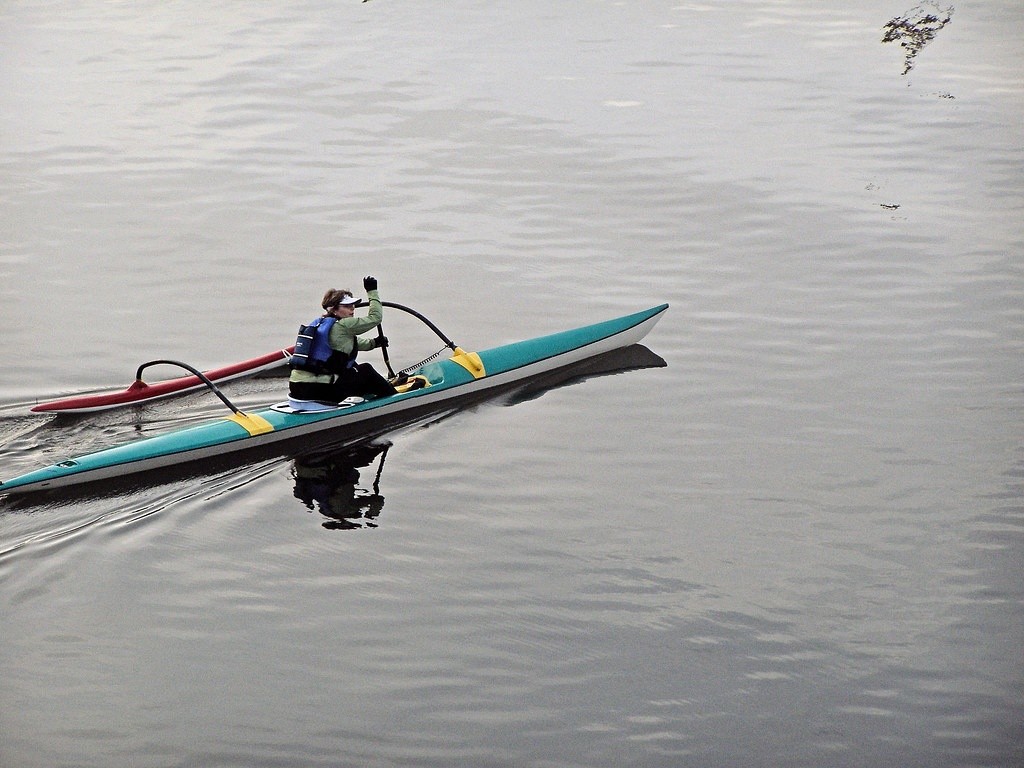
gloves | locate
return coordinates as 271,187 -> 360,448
364,276 -> 377,292
374,337 -> 388,348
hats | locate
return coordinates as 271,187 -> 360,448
326,294 -> 362,312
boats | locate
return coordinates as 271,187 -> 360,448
0,300 -> 671,511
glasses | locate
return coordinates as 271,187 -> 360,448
339,304 -> 354,309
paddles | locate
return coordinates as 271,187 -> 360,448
376,322 -> 396,378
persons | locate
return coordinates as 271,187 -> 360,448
289,276 -> 426,403
